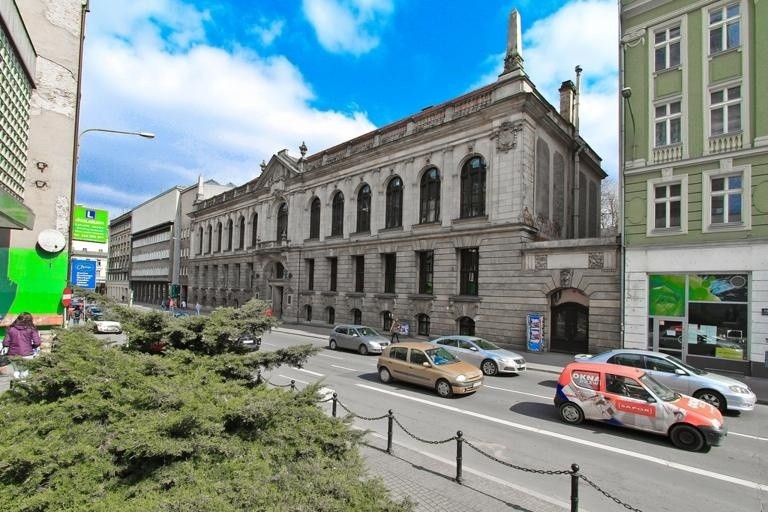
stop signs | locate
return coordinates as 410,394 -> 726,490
60,288 -> 72,307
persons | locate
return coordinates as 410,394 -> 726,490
614,375 -> 632,397
2,311 -> 41,382
73,305 -> 82,324
181,300 -> 186,310
195,301 -> 200,316
389,317 -> 400,343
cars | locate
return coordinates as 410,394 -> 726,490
328,323 -> 391,356
574,348 -> 757,414
553,361 -> 727,453
648,326 -> 742,360
70,294 -> 123,335
377,339 -> 484,398
224,326 -> 262,353
429,334 -> 527,375
